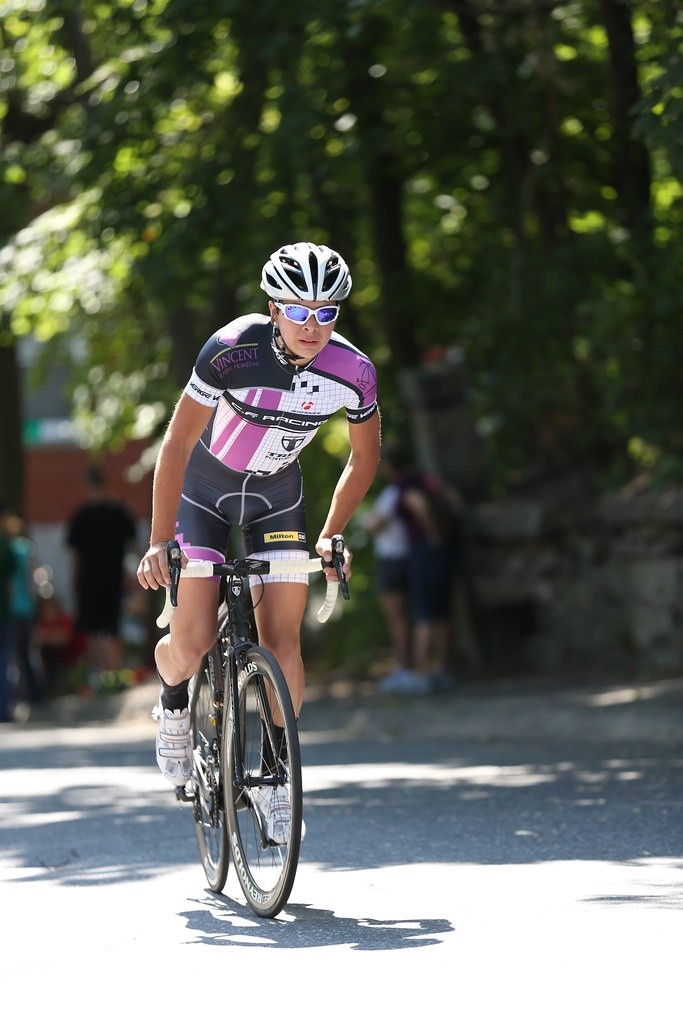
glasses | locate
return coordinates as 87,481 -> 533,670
274,302 -> 340,325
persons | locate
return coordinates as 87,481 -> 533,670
137,242 -> 380,841
359,446 -> 465,695
0,504 -> 43,725
60,464 -> 140,702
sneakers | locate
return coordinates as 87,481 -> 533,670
151,687 -> 194,785
258,785 -> 306,844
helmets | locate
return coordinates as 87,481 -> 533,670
259,242 -> 352,300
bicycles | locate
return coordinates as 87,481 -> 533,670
153,532 -> 352,921
